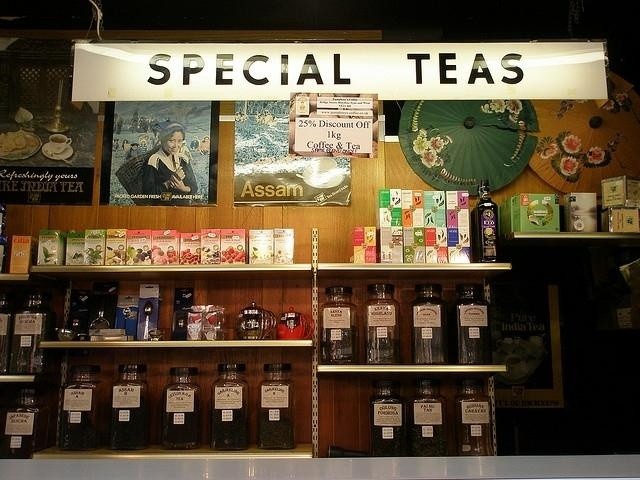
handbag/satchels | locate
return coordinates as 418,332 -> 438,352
115,147 -> 169,206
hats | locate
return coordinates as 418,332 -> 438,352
157,120 -> 186,138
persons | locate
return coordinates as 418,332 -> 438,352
142,119 -> 199,205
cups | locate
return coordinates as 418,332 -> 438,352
48,134 -> 73,154
305,157 -> 339,184
58,328 -> 76,340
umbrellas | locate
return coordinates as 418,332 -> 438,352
526,66 -> 639,195
398,100 -> 539,198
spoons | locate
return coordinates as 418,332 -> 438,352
122,306 -> 132,333
142,299 -> 154,340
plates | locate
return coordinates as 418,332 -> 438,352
0,132 -> 43,160
42,143 -> 73,161
302,171 -> 344,189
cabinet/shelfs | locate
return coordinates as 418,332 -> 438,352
29,260 -> 317,459
0,269 -> 40,386
314,257 -> 522,378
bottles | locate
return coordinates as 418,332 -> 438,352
1,205 -> 9,273
278,306 -> 316,340
55,360 -> 297,451
0,291 -> 57,376
89,309 -> 109,329
454,281 -> 491,365
368,377 -> 493,458
236,301 -> 277,340
409,282 -> 449,365
470,178 -> 500,262
4,387 -> 50,458
320,284 -> 361,365
363,283 -> 401,366
